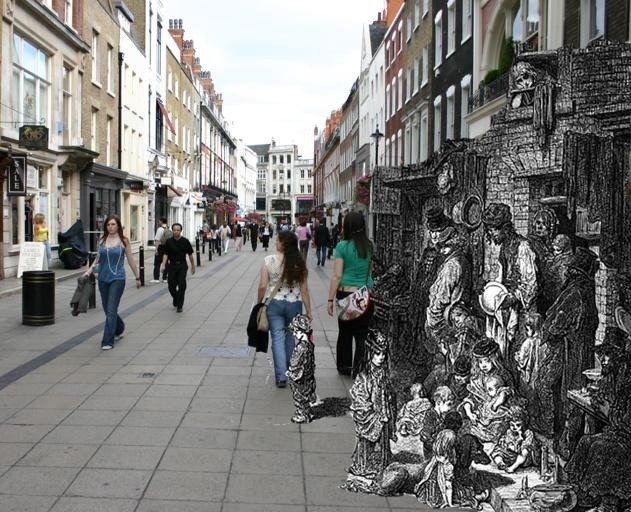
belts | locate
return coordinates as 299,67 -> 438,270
337,285 -> 361,293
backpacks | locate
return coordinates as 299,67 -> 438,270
159,225 -> 173,246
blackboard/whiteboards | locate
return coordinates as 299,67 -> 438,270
17,242 -> 44,276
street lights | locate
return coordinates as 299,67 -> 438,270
368,123 -> 384,165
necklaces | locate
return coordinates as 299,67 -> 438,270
105,234 -> 122,276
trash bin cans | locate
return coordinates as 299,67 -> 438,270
21,271 -> 55,326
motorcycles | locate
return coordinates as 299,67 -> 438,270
56,219 -> 89,269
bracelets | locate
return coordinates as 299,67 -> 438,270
327,298 -> 333,304
135,277 -> 140,281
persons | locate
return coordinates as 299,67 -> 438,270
33,211 -> 49,262
346,203 -> 630,512
256,230 -> 313,387
198,218 -> 329,266
78,213 -> 141,351
148,215 -> 170,283
323,211 -> 374,378
283,314 -> 316,424
159,224 -> 195,312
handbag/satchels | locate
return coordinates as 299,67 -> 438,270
256,305 -> 270,332
335,286 -> 372,322
